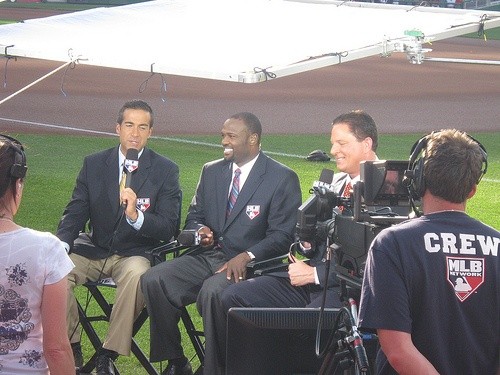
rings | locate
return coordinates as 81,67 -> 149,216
239,277 -> 243,280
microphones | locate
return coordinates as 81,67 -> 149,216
178,230 -> 207,246
312,169 -> 334,195
122,148 -> 139,208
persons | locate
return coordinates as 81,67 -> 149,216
57,101 -> 181,375
221,110 -> 380,375
140,112 -> 302,375
358,128 -> 500,375
384,171 -> 399,194
0,133 -> 77,375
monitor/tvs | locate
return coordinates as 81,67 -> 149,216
226,308 -> 345,375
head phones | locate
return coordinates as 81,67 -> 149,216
403,133 -> 488,201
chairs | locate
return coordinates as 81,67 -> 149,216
74,192 -> 309,375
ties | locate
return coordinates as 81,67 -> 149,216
120,170 -> 127,206
340,182 -> 352,214
226,168 -> 241,218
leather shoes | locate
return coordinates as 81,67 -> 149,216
161,359 -> 194,375
72,345 -> 84,368
95,356 -> 116,375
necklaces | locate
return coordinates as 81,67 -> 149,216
0,219 -> 13,223
